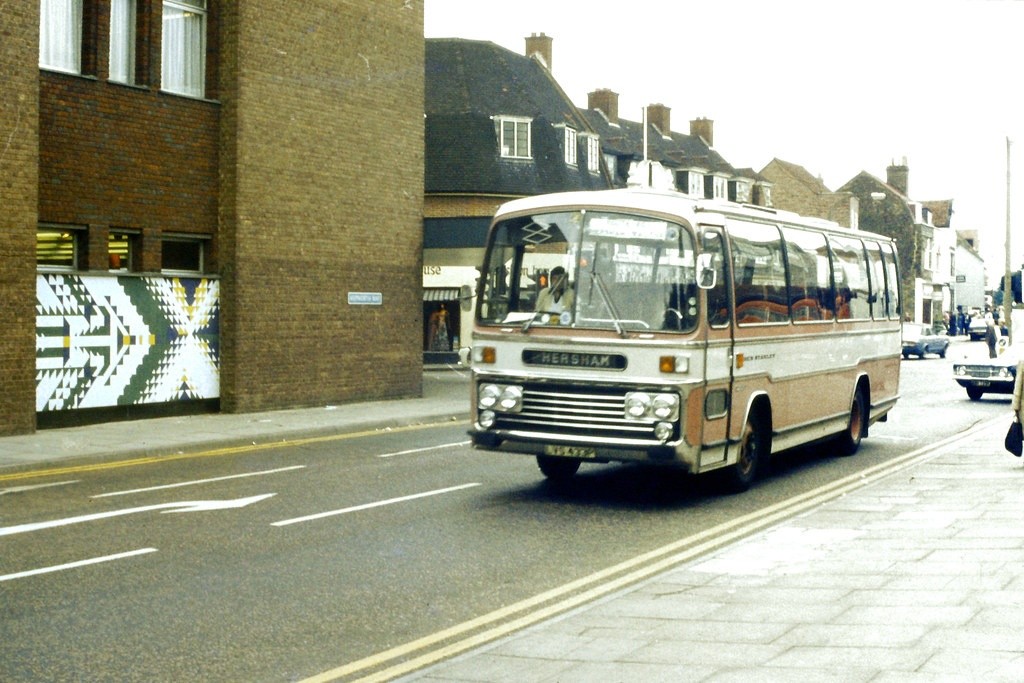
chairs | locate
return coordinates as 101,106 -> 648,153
604,283 -> 889,331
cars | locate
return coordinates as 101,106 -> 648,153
951,338 -> 1024,401
968,315 -> 1007,339
898,322 -> 953,359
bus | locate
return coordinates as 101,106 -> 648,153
459,184 -> 904,489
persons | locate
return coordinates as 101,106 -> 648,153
436,304 -> 451,351
992,308 -> 1000,325
535,266 -> 574,314
943,309 -> 972,336
1012,359 -> 1024,440
984,319 -> 1001,359
